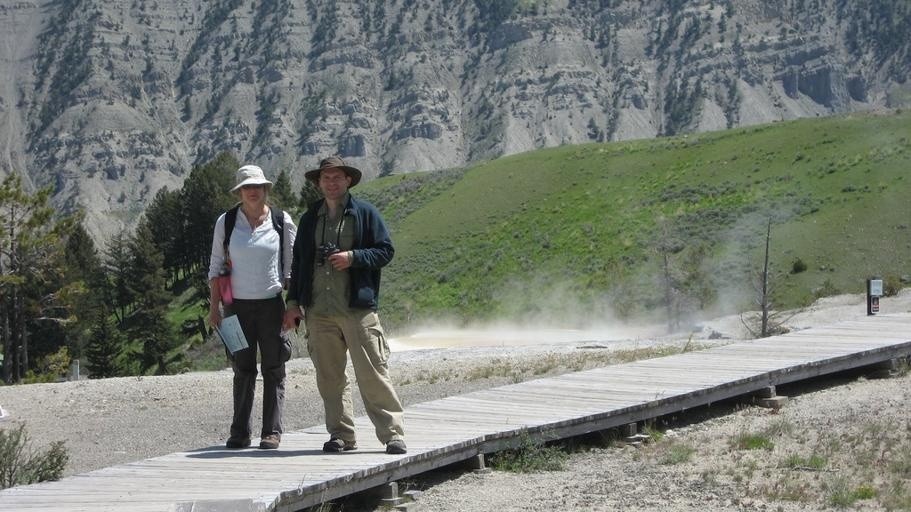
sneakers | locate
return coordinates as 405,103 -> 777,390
386,438 -> 407,454
323,439 -> 357,452
226,436 -> 251,448
260,435 -> 279,449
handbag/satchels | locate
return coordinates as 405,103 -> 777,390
219,267 -> 233,305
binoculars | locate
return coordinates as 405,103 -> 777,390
317,243 -> 340,266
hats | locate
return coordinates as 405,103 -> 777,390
304,156 -> 362,189
229,166 -> 273,195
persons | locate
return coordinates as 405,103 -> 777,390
278,156 -> 408,453
205,164 -> 298,449
